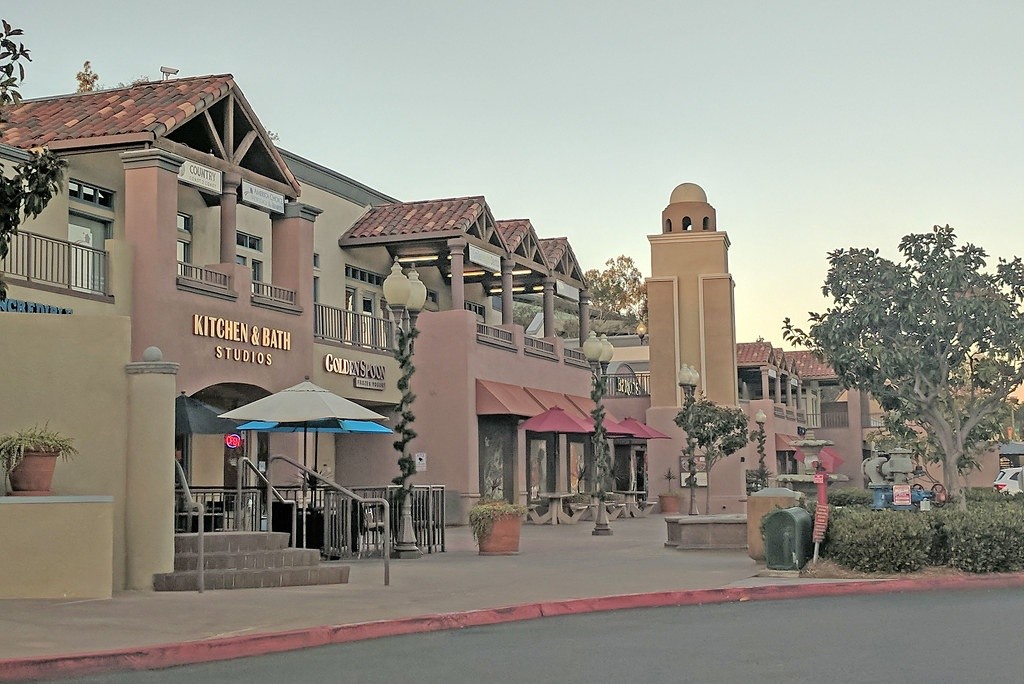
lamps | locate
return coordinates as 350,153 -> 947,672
637,320 -> 649,346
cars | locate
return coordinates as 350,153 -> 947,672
993,468 -> 1023,496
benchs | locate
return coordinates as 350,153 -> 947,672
527,503 -> 601,525
605,501 -> 657,521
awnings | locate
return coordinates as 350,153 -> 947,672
475,378 -> 545,416
563,394 -> 619,424
524,387 -> 586,420
787,435 -> 802,441
775,433 -> 797,451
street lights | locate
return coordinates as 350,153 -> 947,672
384,256 -> 427,560
755,409 -> 766,490
583,331 -> 615,536
678,364 -> 700,516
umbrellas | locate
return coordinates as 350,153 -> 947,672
617,417 -> 671,491
515,403 -> 595,493
215,375 -> 390,486
174,390 -> 241,486
583,413 -> 635,437
237,419 -> 394,508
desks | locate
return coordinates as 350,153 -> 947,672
580,492 -> 613,522
539,493 -> 574,526
613,491 -> 646,518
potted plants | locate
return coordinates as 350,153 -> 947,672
657,468 -> 679,515
469,499 -> 525,556
0,420 -> 81,496
